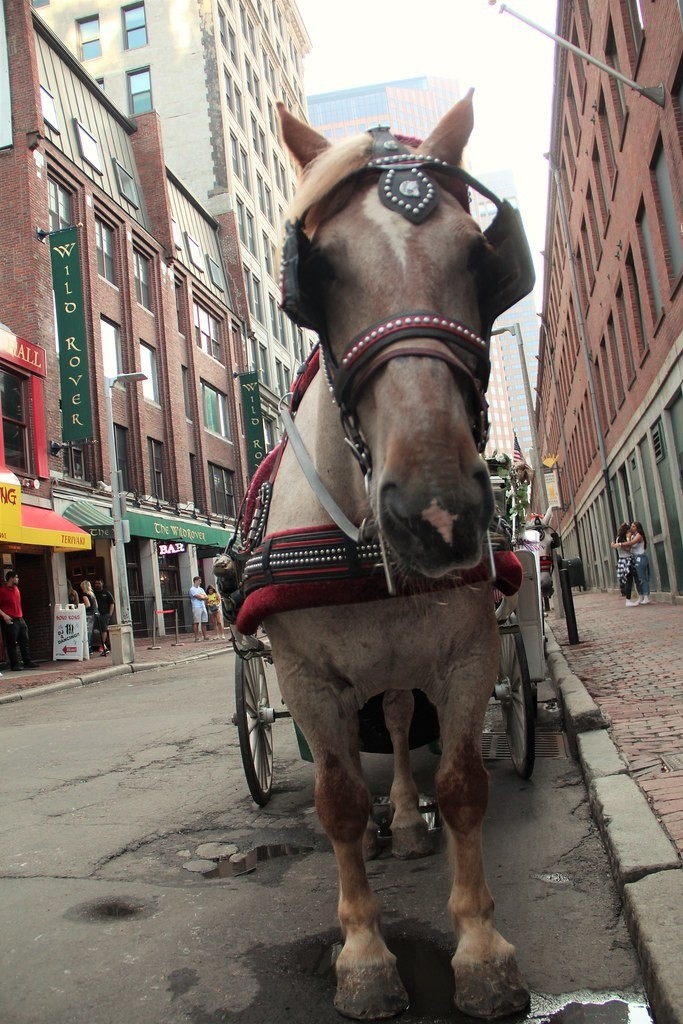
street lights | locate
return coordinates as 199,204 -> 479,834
104,372 -> 148,624
490,323 -> 564,620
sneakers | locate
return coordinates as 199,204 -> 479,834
101,649 -> 109,655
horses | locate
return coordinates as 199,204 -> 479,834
214,88 -> 536,1021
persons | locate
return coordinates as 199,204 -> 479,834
66,579 -> 79,608
0,571 -> 41,671
80,580 -> 98,656
93,579 -> 115,652
188,577 -> 212,642
205,585 -> 226,640
611,521 -> 652,607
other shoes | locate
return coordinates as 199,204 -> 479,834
10,666 -> 21,671
195,637 -> 199,643
636,595 -> 650,605
216,635 -> 225,640
204,637 -> 212,641
626,596 -> 639,607
23,662 -> 40,668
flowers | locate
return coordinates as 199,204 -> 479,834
494,452 -> 529,527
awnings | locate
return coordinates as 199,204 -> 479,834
0,502 -> 93,552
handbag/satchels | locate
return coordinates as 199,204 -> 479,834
210,605 -> 217,611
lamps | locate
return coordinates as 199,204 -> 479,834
168,498 -> 181,516
185,503 -> 197,519
215,513 -> 226,528
126,488 -> 141,508
200,509 -> 211,526
147,493 -> 162,511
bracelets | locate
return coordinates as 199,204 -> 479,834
619,542 -> 622,547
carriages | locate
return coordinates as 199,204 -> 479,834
212,88 -> 547,1019
